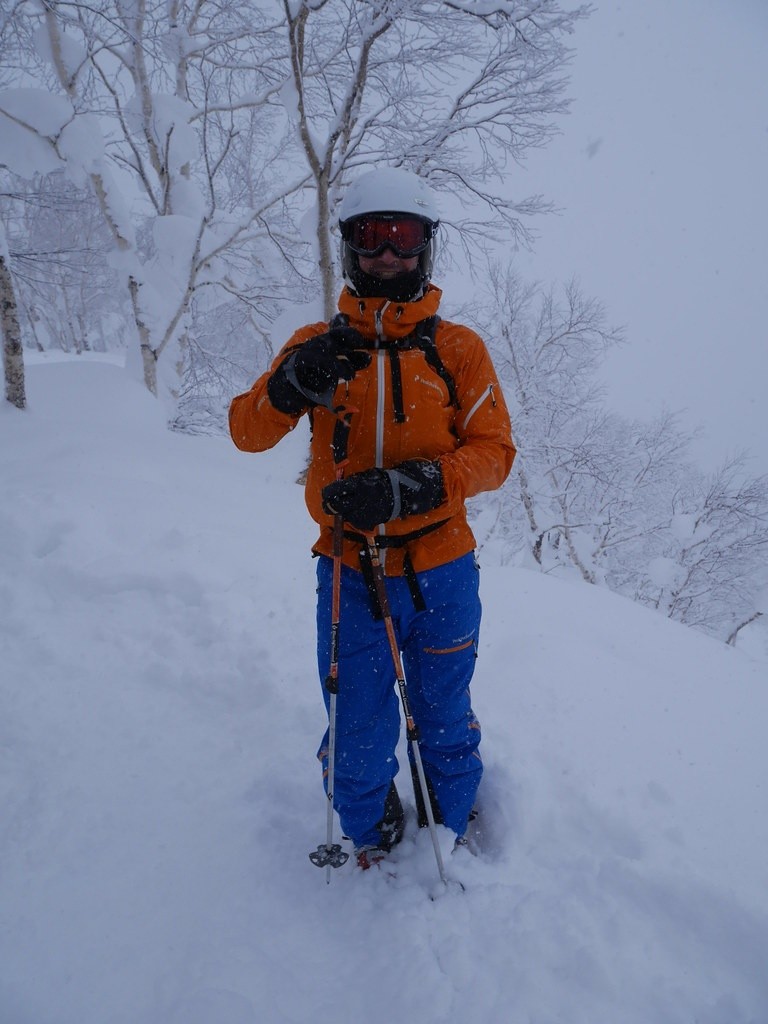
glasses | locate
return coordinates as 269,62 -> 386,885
345,216 -> 431,257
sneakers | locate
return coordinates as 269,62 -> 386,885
353,778 -> 405,870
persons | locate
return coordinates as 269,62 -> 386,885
229,168 -> 514,869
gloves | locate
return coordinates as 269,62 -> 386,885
265,326 -> 371,417
320,457 -> 449,535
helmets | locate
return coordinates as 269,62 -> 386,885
339,167 -> 440,299
